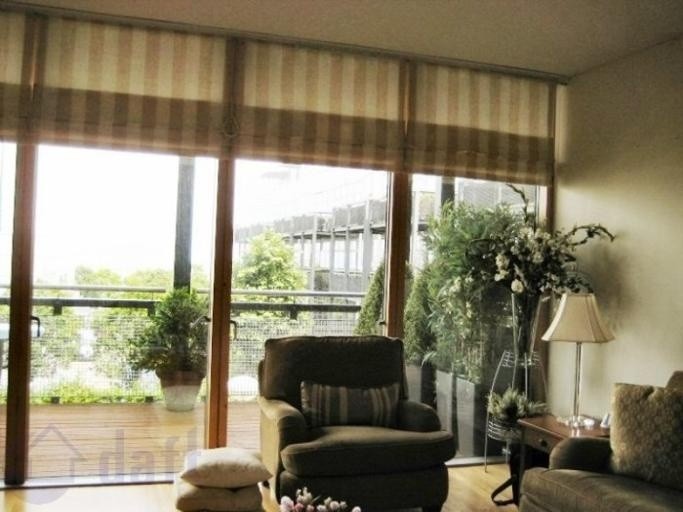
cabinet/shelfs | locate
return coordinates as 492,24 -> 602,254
516,415 -> 610,483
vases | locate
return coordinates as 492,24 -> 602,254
510,291 -> 540,399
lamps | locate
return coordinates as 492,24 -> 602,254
541,292 -> 615,427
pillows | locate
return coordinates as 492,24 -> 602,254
180,447 -> 274,487
610,383 -> 683,482
300,380 -> 399,427
175,474 -> 262,512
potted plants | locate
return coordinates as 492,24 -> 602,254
126,285 -> 212,411
404,199 -> 535,458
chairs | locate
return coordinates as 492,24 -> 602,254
258,335 -> 456,512
520,370 -> 682,511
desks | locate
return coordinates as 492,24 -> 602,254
487,418 -> 523,505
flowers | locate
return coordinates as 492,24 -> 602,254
470,183 -> 615,391
279,487 -> 361,511
